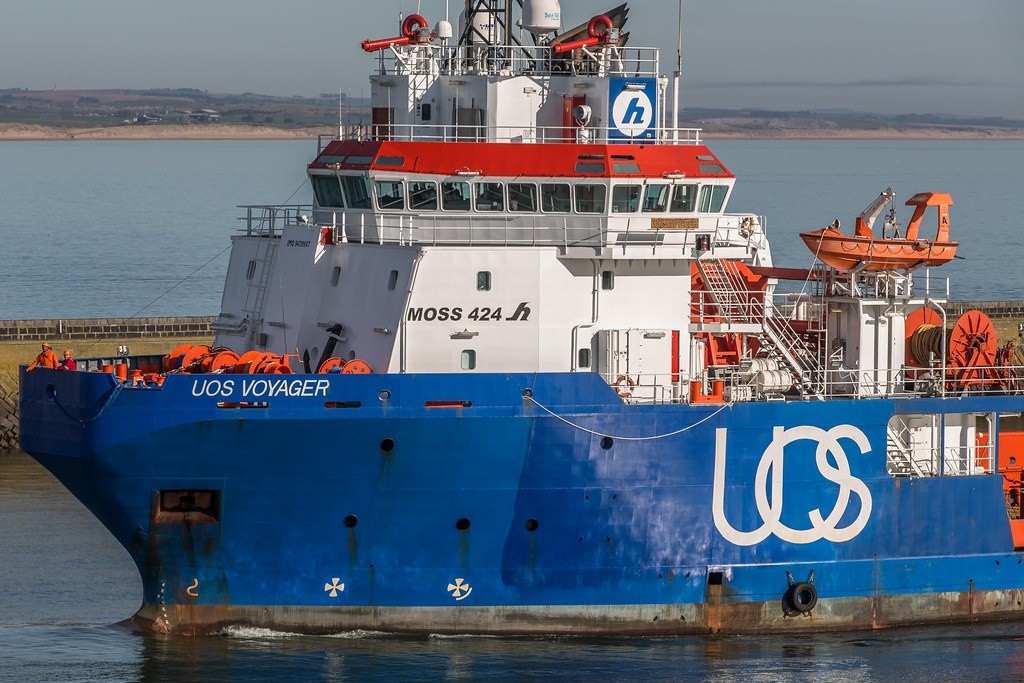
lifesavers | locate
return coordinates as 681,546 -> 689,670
787,581 -> 818,613
616,376 -> 634,397
741,218 -> 755,238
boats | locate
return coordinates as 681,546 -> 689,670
18,0 -> 1024,638
800,188 -> 958,271
